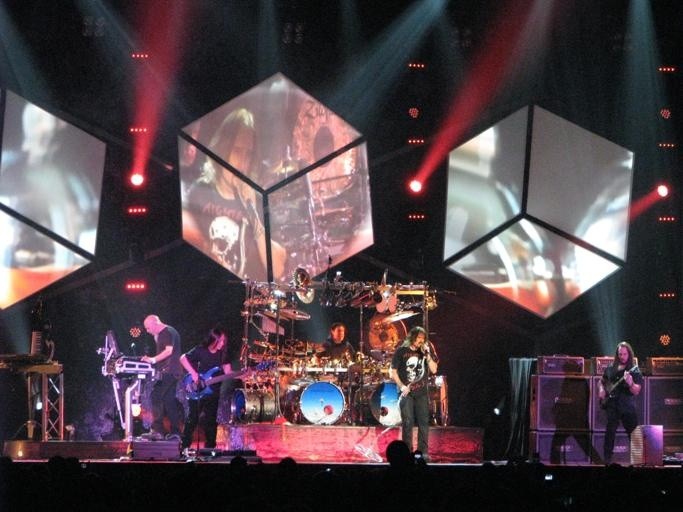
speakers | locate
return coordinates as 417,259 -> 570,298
528,374 -> 593,430
529,432 -> 592,465
593,429 -> 633,465
648,377 -> 683,433
592,375 -> 647,432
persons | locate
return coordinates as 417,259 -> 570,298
597,341 -> 644,466
179,328 -> 252,461
316,322 -> 356,362
1,440 -> 682,511
140,315 -> 183,441
182,108 -> 286,282
390,326 -> 438,462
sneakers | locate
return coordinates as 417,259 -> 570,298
141,432 -> 161,440
164,434 -> 179,441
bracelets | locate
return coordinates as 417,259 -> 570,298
401,385 -> 407,391
150,358 -> 156,365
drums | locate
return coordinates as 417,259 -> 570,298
292,379 -> 346,426
426,374 -> 448,427
230,389 -> 277,423
354,381 -> 402,426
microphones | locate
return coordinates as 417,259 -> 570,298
30,297 -> 43,316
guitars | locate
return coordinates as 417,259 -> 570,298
182,359 -> 275,400
599,365 -> 639,408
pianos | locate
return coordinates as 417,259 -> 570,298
30,331 -> 42,355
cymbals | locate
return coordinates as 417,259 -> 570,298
280,308 -> 310,322
